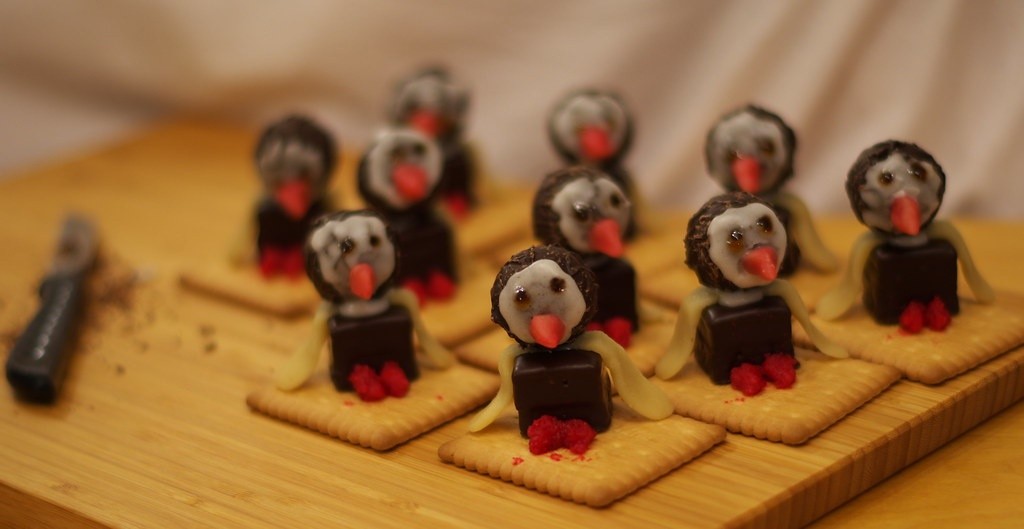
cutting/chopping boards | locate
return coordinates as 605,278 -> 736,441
1,130 -> 1024,529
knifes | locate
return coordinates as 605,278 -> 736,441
7,213 -> 101,403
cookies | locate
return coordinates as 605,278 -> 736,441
174,179 -> 1024,507
